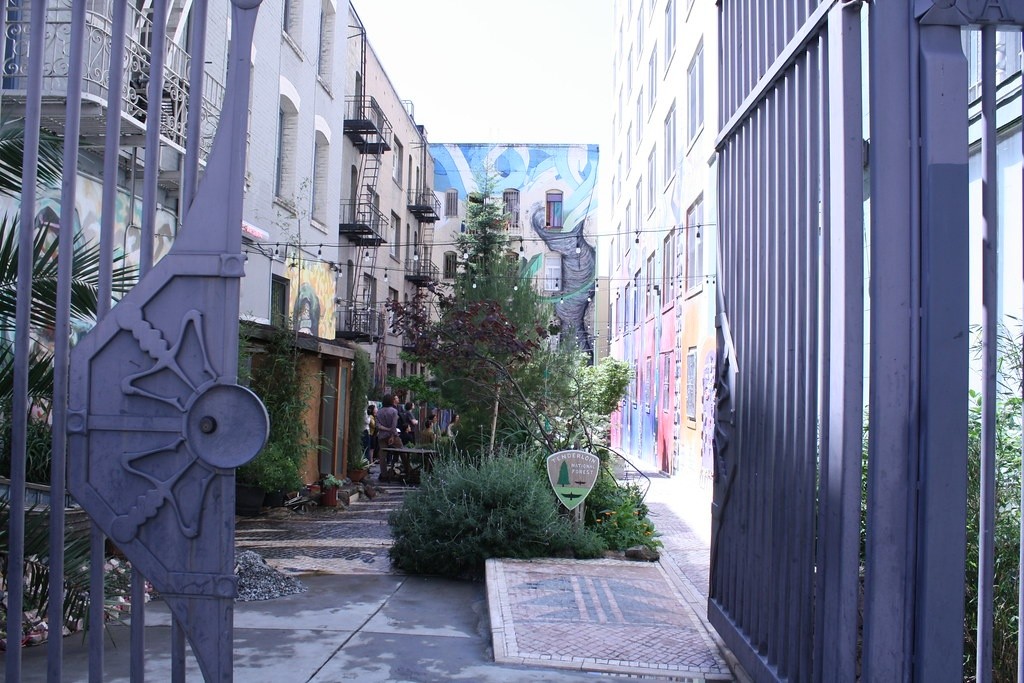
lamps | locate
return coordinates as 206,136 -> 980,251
653,284 -> 661,296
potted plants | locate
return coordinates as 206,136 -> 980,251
236,220 -> 338,516
349,444 -> 378,481
323,474 -> 340,506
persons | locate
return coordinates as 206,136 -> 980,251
387,394 -> 418,464
424,415 -> 441,436
447,414 -> 461,437
376,394 -> 407,473
361,405 -> 379,464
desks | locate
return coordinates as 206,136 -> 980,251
383,447 -> 437,483
